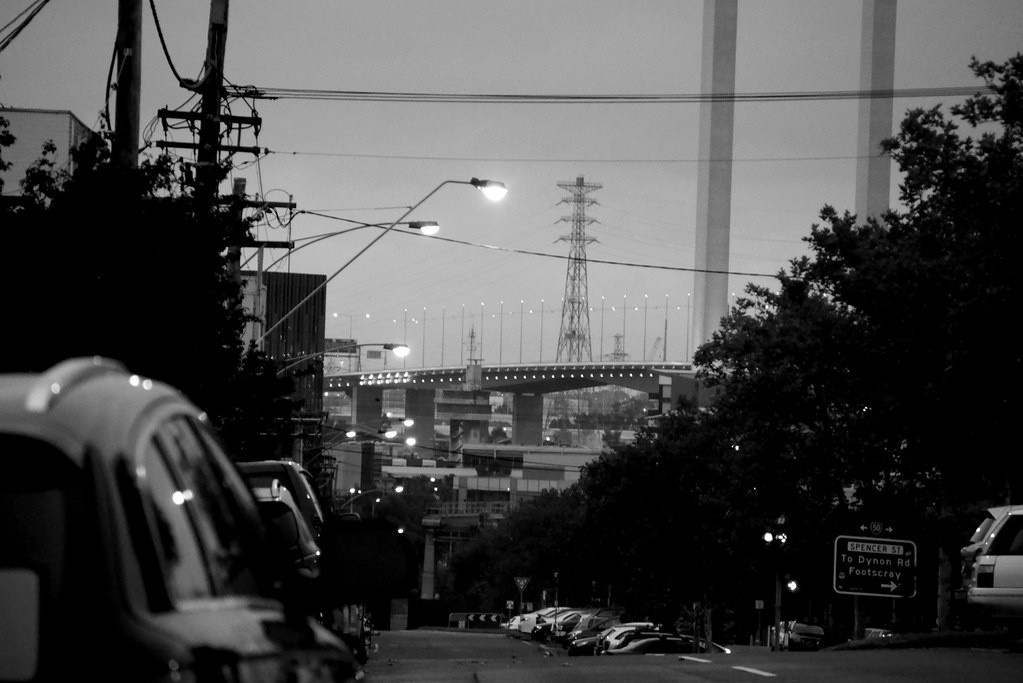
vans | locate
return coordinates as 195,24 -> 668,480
770,620 -> 826,651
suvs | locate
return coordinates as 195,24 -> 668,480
232,457 -> 376,666
953,504 -> 1023,619
0,354 -> 366,683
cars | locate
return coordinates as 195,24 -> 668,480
848,627 -> 893,642
502,606 -> 731,658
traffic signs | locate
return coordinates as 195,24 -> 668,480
859,521 -> 894,536
833,535 -> 919,599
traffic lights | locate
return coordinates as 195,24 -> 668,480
784,579 -> 798,593
761,531 -> 787,544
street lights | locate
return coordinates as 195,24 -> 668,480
249,217 -> 440,370
223,174 -> 504,377
